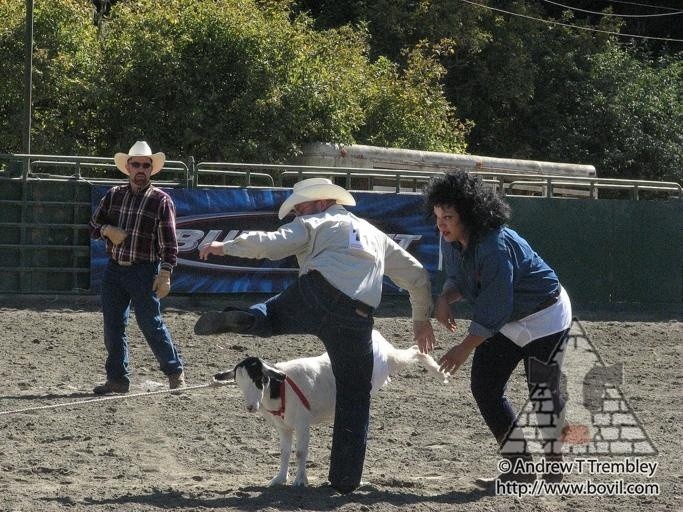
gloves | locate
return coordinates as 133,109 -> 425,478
103,224 -> 127,246
153,270 -> 170,299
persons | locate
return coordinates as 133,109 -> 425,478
425,167 -> 572,496
89,139 -> 187,394
193,177 -> 437,494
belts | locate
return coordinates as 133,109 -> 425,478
116,261 -> 150,267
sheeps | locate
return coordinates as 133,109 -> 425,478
212,329 -> 452,488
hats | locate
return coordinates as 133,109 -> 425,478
113,140 -> 166,176
277,178 -> 356,221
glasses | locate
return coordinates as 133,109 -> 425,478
293,204 -> 310,216
129,162 -> 152,168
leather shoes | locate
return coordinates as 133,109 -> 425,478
542,454 -> 563,484
193,310 -> 255,336
475,452 -> 537,492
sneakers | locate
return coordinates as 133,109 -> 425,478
168,370 -> 186,394
93,381 -> 130,395
